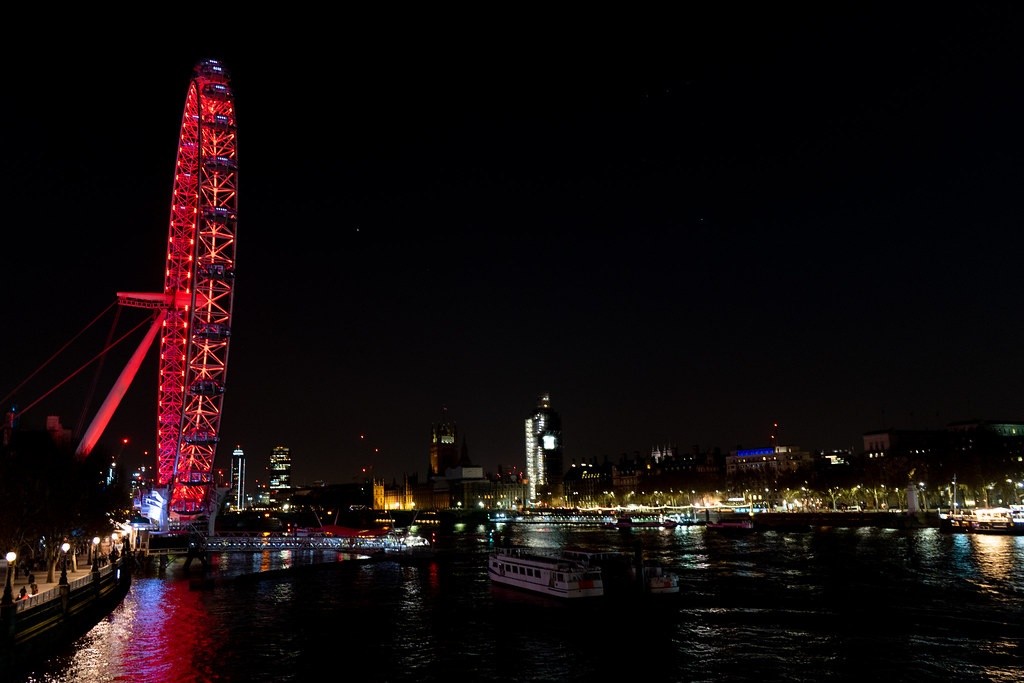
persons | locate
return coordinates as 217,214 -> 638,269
19,577 -> 39,606
705,509 -> 710,521
20,549 -> 48,578
98,548 -> 120,567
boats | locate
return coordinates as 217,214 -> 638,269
704,517 -> 757,531
940,516 -> 975,534
487,547 -> 605,598
639,558 -> 681,594
562,546 -> 636,575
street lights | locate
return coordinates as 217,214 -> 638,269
58,543 -> 70,584
1,551 -> 17,606
91,536 -> 101,573
110,533 -> 120,564
